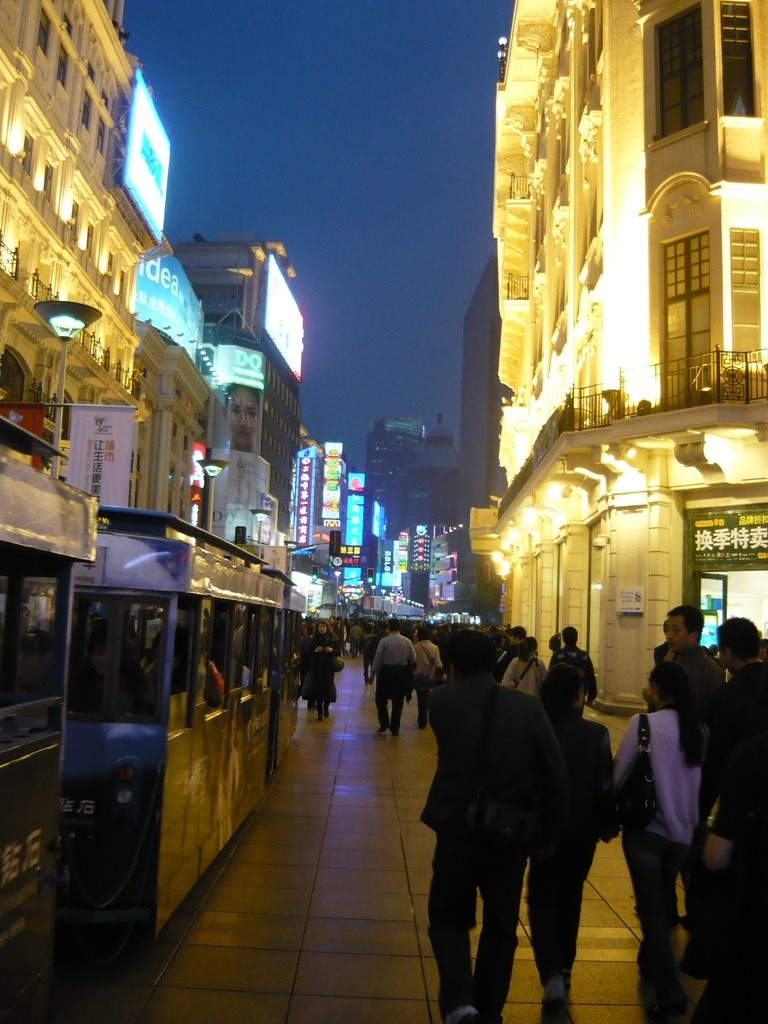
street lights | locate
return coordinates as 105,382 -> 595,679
250,508 -> 274,557
370,584 -> 376,619
284,540 -> 297,577
390,593 -> 424,620
34,299 -> 102,480
381,589 -> 386,614
334,570 -> 342,617
196,459 -> 233,534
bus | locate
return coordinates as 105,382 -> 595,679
0,419 -> 101,1024
37,504 -> 306,944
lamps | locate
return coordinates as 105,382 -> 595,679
144,318 -> 231,399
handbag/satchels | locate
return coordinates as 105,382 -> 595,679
344,642 -> 351,650
620,712 -> 657,831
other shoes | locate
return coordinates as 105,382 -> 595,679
445,1004 -> 503,1024
323,704 -> 329,718
317,714 -> 322,721
541,970 -> 567,1024
376,726 -> 389,733
392,732 -> 399,736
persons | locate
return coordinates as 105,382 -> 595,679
299,605 -> 768,1024
225,384 -> 262,452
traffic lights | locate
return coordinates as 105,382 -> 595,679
367,568 -> 375,583
311,565 -> 319,581
